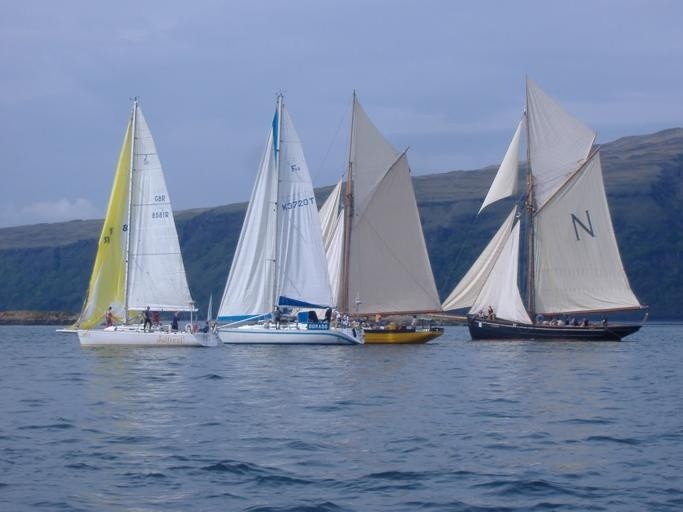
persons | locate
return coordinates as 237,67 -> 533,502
411,315 -> 417,331
478,310 -> 484,319
198,321 -> 209,333
144,306 -> 153,333
536,313 -> 608,327
106,306 -> 112,327
331,309 -> 337,329
273,306 -> 282,330
488,306 -> 493,321
171,312 -> 178,331
322,308 -> 332,329
